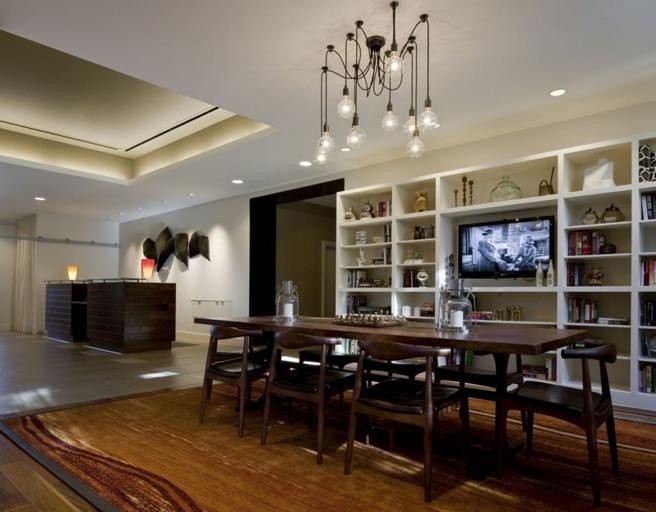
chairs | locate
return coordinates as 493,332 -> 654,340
300,337 -> 363,429
437,347 -> 529,435
259,330 -> 358,466
507,341 -> 621,506
364,344 -> 439,383
433,318 -> 528,435
197,323 -> 273,440
344,335 -> 469,506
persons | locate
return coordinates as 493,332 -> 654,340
507,235 -> 536,271
477,229 -> 508,272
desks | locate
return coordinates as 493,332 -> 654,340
190,312 -> 589,480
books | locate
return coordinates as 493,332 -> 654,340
566,192 -> 656,393
346,199 -> 435,356
453,347 -> 556,382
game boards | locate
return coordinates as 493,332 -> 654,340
331,319 -> 408,327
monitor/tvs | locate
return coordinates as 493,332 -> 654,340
458,215 -> 555,279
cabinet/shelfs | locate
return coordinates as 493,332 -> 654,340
335,130 -> 656,413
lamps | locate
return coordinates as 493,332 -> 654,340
141,259 -> 155,281
314,0 -> 439,165
67,266 -> 78,281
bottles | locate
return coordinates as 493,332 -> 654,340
547,259 -> 556,287
274,280 -> 299,322
490,175 -> 522,202
434,279 -> 477,334
535,258 -> 545,287
